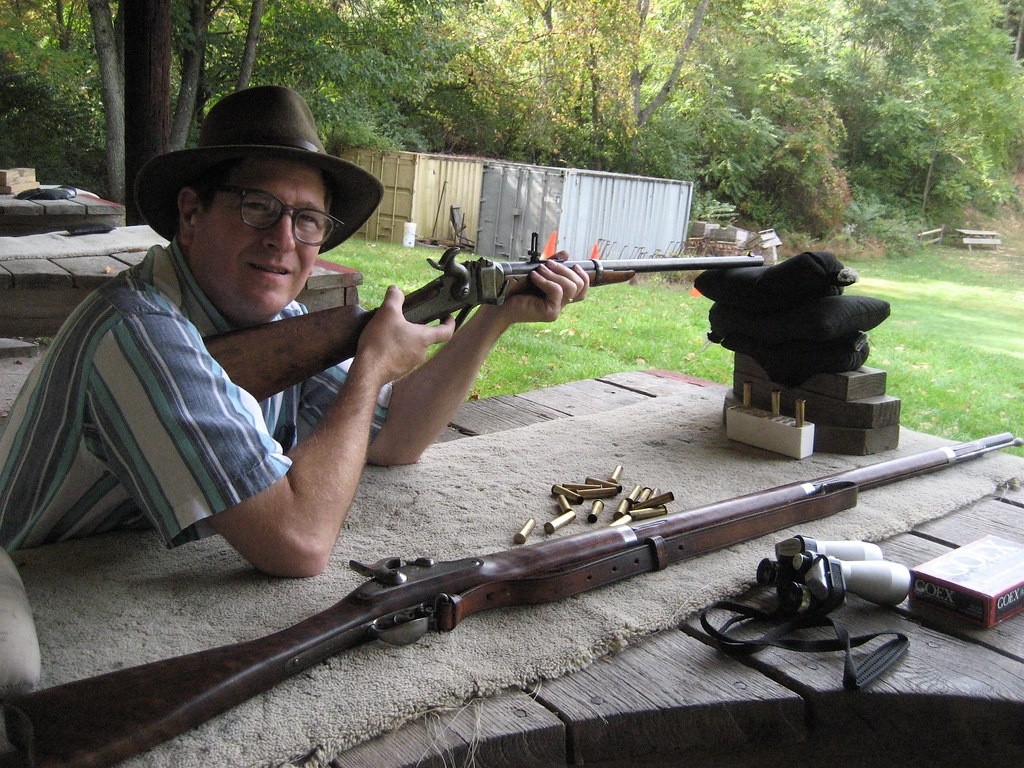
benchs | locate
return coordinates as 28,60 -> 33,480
952,236 -> 1002,252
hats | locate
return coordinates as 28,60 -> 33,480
133,85 -> 384,254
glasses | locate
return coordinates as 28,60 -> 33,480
216,184 -> 345,247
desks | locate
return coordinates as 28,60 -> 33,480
0,185 -> 127,237
954,229 -> 1001,252
0,368 -> 1023,768
0,225 -> 364,337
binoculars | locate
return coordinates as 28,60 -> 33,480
756,533 -> 912,614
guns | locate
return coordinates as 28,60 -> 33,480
1,430 -> 1024,767
195,230 -> 767,405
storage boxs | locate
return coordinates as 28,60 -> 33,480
907,535 -> 1023,630
340,146 -> 695,266
694,222 -> 782,251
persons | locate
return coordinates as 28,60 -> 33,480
0,87 -> 590,579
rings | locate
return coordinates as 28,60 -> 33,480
564,297 -> 574,303
